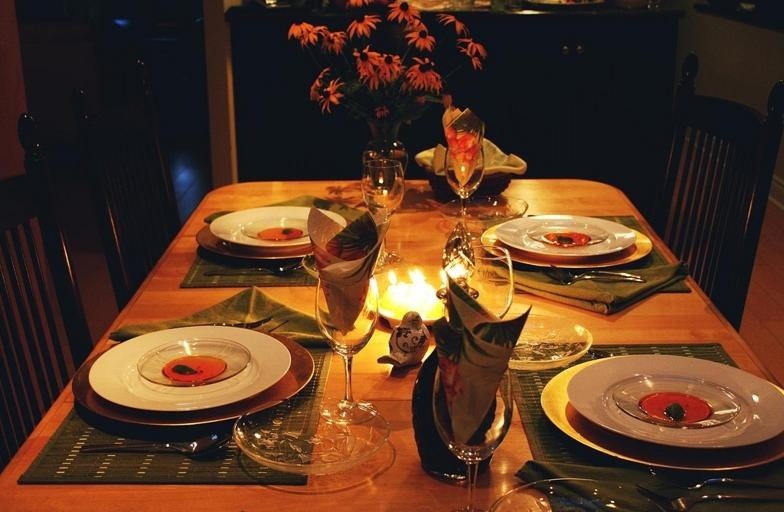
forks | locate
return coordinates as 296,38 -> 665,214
648,465 -> 780,489
632,482 -> 780,511
545,267 -> 645,286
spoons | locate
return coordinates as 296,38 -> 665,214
79,428 -> 235,458
202,261 -> 306,279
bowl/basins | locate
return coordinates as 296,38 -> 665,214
418,163 -> 513,204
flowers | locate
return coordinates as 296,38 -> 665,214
287,0 -> 487,138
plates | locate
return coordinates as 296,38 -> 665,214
88,324 -> 291,414
494,212 -> 638,263
441,195 -> 529,223
232,392 -> 391,477
208,205 -> 347,249
540,354 -> 780,472
498,313 -> 593,371
71,330 -> 316,430
566,352 -> 780,451
301,253 -> 324,281
487,477 -> 665,511
480,223 -> 654,270
196,226 -> 319,261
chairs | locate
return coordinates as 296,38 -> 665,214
0,113 -> 95,477
76,56 -> 181,314
643,53 -> 783,332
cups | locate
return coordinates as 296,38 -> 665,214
457,242 -> 514,322
430,365 -> 514,512
360,160 -> 405,266
315,273 -> 379,426
442,144 -> 487,240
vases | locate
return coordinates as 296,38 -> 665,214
362,138 -> 410,174
